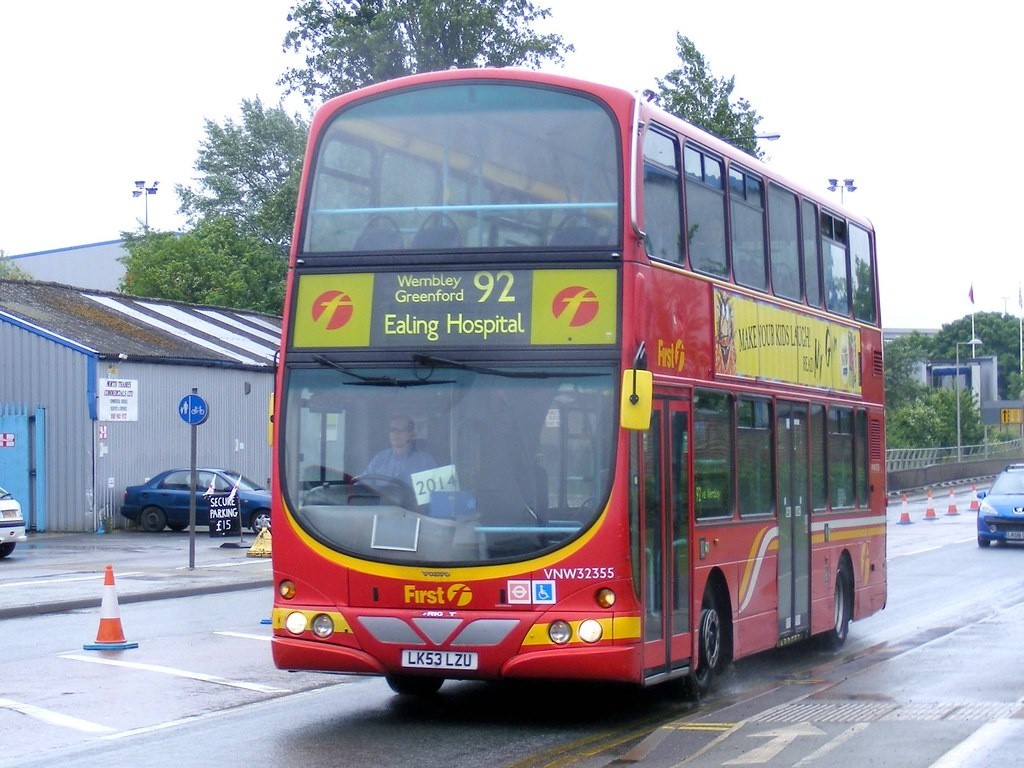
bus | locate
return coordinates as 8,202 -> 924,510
267,67 -> 888,705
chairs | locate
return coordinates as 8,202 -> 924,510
773,262 -> 793,296
205,479 -> 223,491
351,216 -> 403,250
199,480 -> 206,491
735,259 -> 763,287
546,213 -> 611,247
414,439 -> 441,472
412,212 -> 461,249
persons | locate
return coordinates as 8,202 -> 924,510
357,415 -> 441,490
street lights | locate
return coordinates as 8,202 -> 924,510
131,179 -> 159,223
956,339 -> 983,460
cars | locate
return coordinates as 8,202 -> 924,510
120,466 -> 271,536
0,486 -> 28,558
976,462 -> 1024,547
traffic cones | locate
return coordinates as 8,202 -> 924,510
82,563 -> 139,651
966,482 -> 981,511
896,494 -> 915,525
942,487 -> 961,516
922,490 -> 940,519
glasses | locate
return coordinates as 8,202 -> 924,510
389,426 -> 405,432
